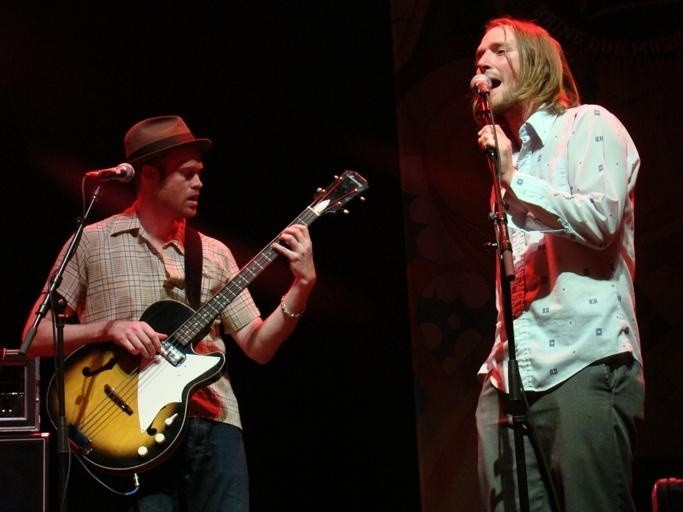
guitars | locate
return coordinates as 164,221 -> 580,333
46,166 -> 370,476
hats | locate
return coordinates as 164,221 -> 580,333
124,116 -> 211,166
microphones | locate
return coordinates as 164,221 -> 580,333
469,74 -> 492,97
84,163 -> 135,183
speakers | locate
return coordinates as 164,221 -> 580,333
0,432 -> 52,512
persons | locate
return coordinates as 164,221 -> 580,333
468,13 -> 649,512
15,115 -> 317,512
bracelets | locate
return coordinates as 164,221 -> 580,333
279,295 -> 307,319
